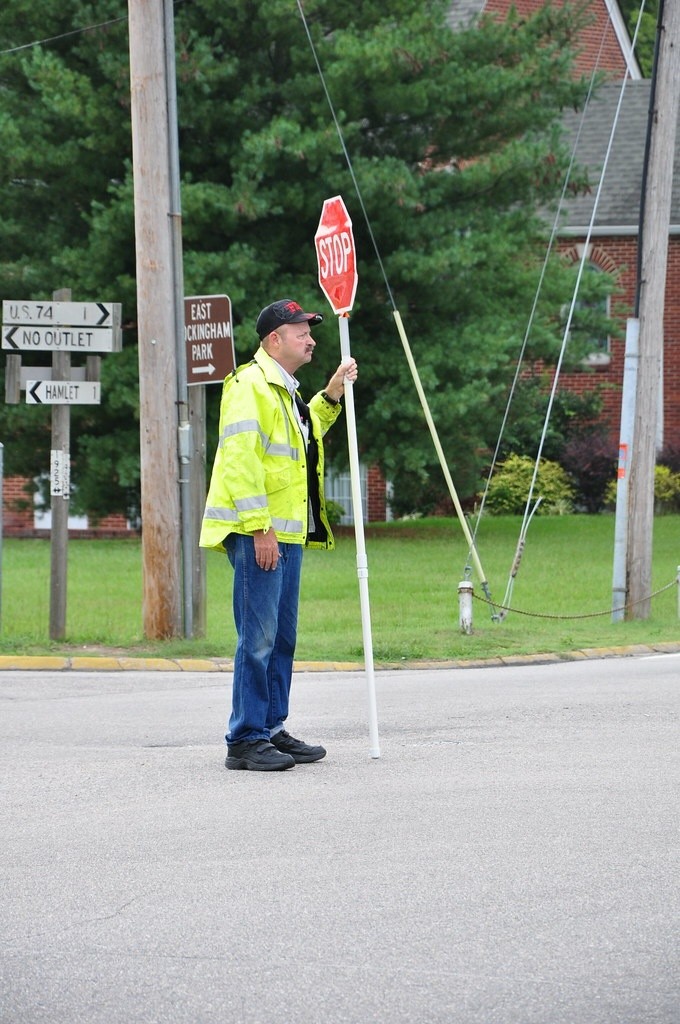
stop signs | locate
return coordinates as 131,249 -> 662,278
314,194 -> 359,315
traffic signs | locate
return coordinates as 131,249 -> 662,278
0,325 -> 123,354
24,379 -> 101,406
1,299 -> 123,328
184,294 -> 236,386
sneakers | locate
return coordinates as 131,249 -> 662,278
224,731 -> 326,773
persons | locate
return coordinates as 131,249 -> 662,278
199,299 -> 358,772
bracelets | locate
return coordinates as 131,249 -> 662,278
321,392 -> 337,406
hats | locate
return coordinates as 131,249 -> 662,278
256,299 -> 323,341
